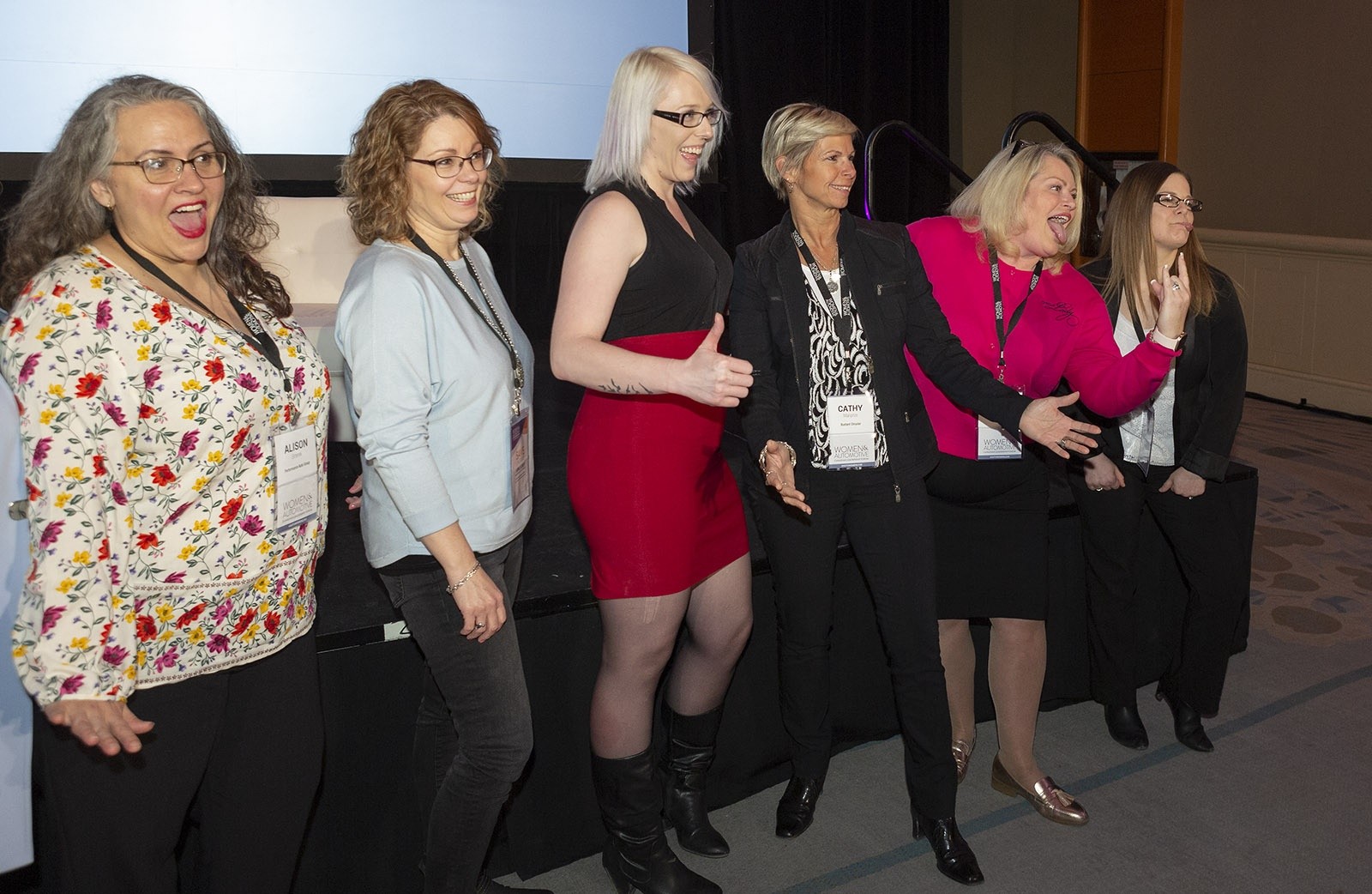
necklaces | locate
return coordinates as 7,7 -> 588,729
442,244 -> 526,388
813,242 -> 837,294
174,261 -> 222,328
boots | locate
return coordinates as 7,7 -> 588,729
591,743 -> 724,894
658,699 -> 731,858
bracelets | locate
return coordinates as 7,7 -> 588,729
446,561 -> 480,594
759,441 -> 797,472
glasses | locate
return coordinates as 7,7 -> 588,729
403,148 -> 493,178
101,152 -> 228,184
1151,193 -> 1205,212
1008,138 -> 1041,165
652,109 -> 722,128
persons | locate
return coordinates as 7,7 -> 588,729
904,138 -> 1193,824
549,45 -> 755,894
0,75 -> 329,894
1052,161 -> 1248,752
335,78 -> 553,894
728,102 -> 1101,886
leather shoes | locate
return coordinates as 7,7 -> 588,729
952,726 -> 977,785
990,752 -> 1090,827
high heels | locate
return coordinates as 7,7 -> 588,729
1154,677 -> 1215,752
1100,685 -> 1148,750
909,800 -> 984,886
775,775 -> 824,839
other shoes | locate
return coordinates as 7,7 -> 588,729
477,874 -> 552,894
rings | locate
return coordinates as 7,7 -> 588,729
1061,436 -> 1069,449
475,623 -> 487,630
1096,487 -> 1104,491
766,471 -> 776,480
781,481 -> 787,486
1188,496 -> 1193,500
1172,282 -> 1179,290
